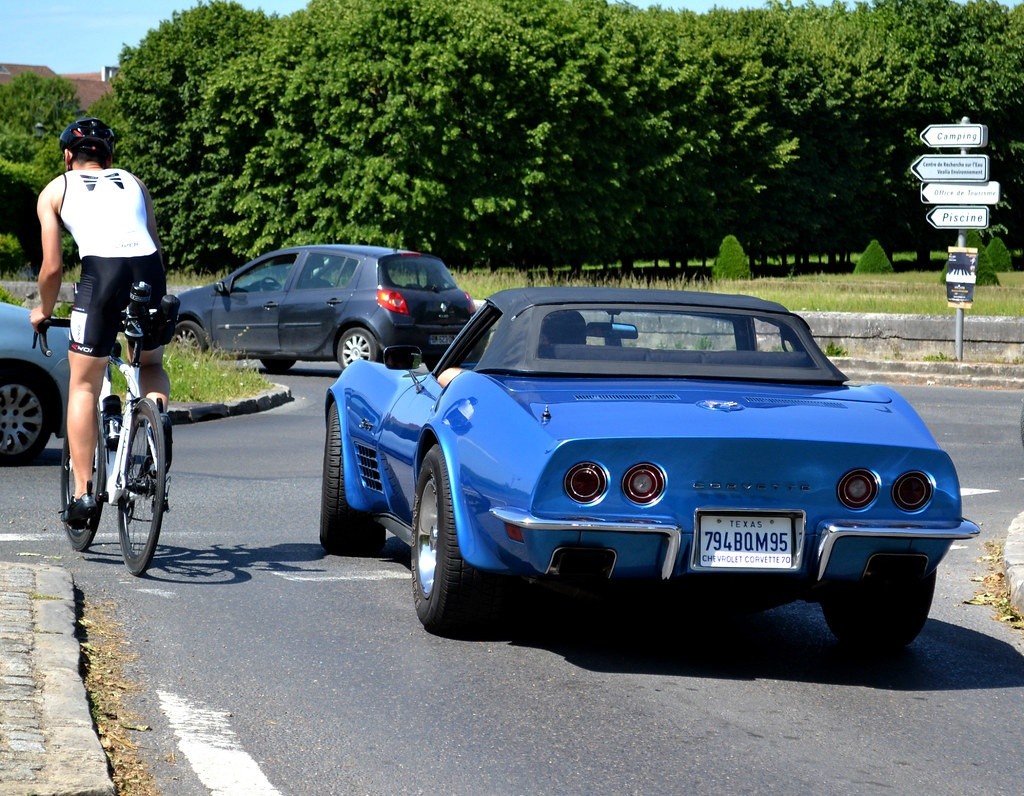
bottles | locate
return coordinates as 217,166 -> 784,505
101,395 -> 125,450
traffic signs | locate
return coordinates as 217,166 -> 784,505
920,180 -> 1001,204
919,123 -> 988,148
926,206 -> 990,229
910,154 -> 991,184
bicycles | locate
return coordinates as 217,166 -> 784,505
33,279 -> 181,576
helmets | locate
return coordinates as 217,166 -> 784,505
59,117 -> 116,157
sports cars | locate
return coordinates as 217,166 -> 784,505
318,288 -> 982,656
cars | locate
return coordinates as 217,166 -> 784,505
0,302 -> 72,467
172,244 -> 475,371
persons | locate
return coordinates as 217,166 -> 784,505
29,117 -> 173,522
438,310 -> 587,391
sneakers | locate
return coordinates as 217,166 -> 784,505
145,398 -> 172,478
58,480 -> 97,522
